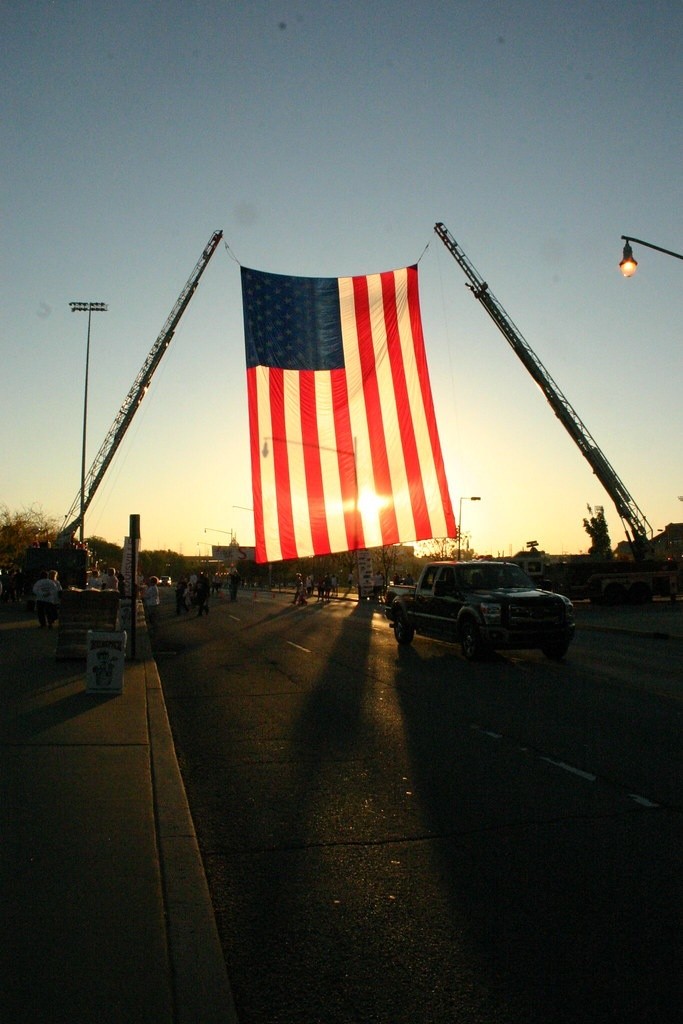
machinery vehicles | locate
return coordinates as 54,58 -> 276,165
431,220 -> 683,612
14,229 -> 226,608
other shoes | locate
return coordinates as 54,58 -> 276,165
205,609 -> 209,615
48,625 -> 53,630
38,624 -> 47,629
197,613 -> 202,617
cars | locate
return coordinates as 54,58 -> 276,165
159,575 -> 172,587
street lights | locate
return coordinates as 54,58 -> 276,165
204,528 -> 233,541
457,496 -> 482,560
69,301 -> 109,544
261,436 -> 359,592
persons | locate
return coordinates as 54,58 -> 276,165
32,569 -> 63,629
348,571 -> 353,590
228,570 -> 241,602
316,572 -> 338,603
393,571 -> 414,586
374,571 -> 386,604
174,570 -> 223,618
290,573 -> 314,606
140,576 -> 160,633
87,567 -> 119,590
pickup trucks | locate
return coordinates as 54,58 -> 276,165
384,560 -> 578,663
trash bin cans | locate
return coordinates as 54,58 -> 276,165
571,585 -> 585,599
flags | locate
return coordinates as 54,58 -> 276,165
240,263 -> 457,563
